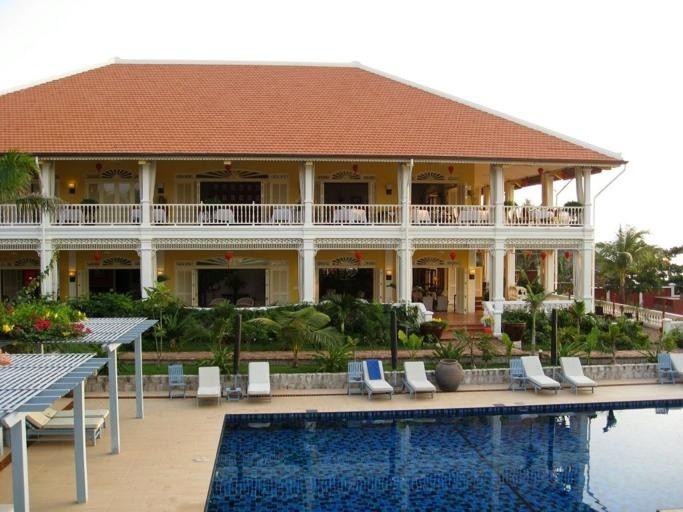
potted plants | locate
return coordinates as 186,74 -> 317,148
419,320 -> 448,343
501,316 -> 526,341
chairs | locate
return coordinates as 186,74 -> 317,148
23,408 -> 109,446
168,360 -> 272,407
657,352 -> 683,384
506,355 -> 599,395
208,297 -> 255,308
343,358 -> 437,401
423,295 -> 448,312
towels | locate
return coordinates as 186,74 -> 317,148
366,359 -> 382,380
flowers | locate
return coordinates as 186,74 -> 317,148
481,316 -> 492,327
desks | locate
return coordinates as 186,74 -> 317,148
133,208 -> 167,222
391,208 -> 429,222
449,209 -> 489,222
56,207 -> 87,224
197,210 -> 235,223
330,208 -> 368,223
505,208 -> 573,222
268,209 -> 293,225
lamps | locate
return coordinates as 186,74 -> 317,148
69,271 -> 75,282
469,270 -> 476,279
386,271 -> 392,280
68,183 -> 75,193
385,183 -> 392,194
157,183 -> 164,193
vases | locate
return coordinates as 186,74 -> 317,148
484,327 -> 491,335
